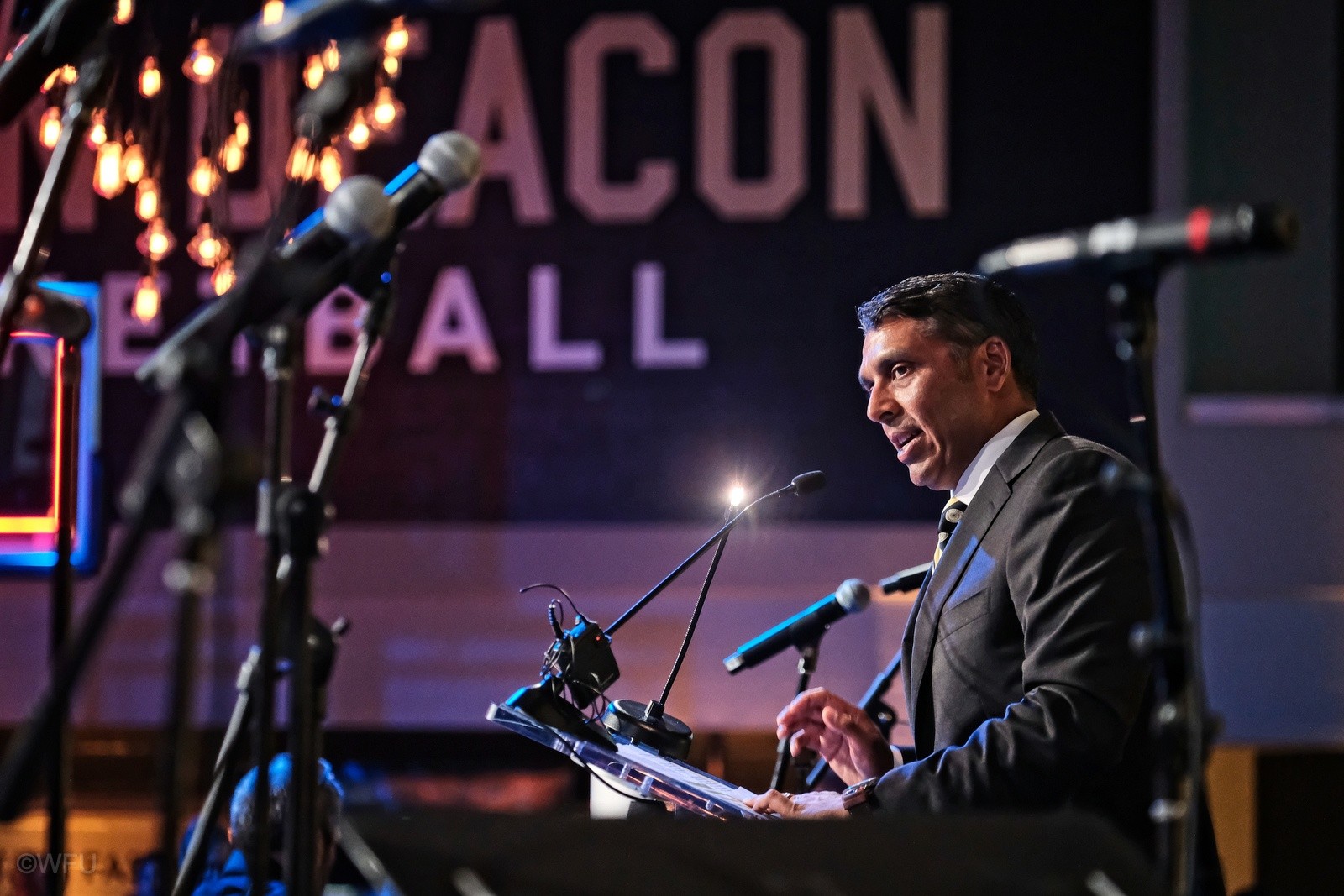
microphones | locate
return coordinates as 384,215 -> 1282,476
232,0 -> 402,60
724,578 -> 871,674
984,203 -> 1289,283
505,469 -> 826,752
135,131 -> 486,397
878,563 -> 933,596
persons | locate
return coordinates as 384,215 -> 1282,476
739,273 -> 1179,896
207,752 -> 342,896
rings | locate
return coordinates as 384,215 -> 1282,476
783,792 -> 793,798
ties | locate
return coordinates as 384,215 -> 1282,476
934,497 -> 967,570
